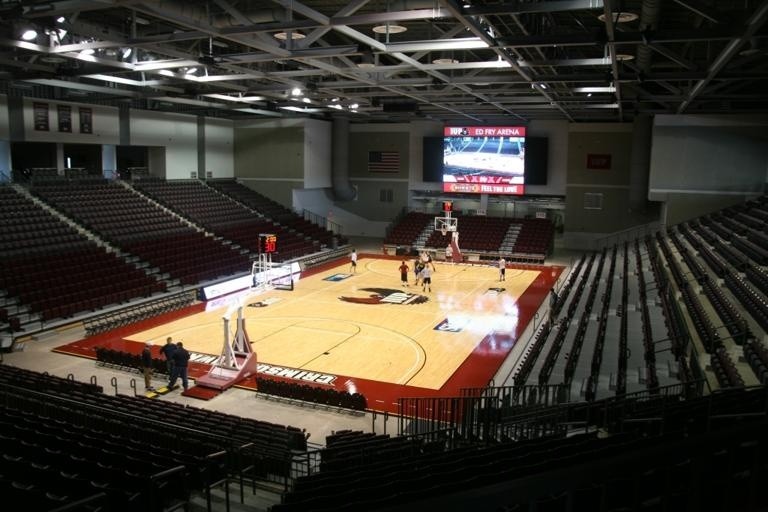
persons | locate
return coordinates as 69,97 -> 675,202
399,261 -> 409,287
167,342 -> 190,391
159,337 -> 177,380
446,244 -> 453,265
141,345 -> 155,391
496,256 -> 506,282
414,250 -> 436,292
549,288 -> 558,316
350,248 -> 357,273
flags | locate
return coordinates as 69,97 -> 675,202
367,150 -> 401,173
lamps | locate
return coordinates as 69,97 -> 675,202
117,46 -> 134,61
273,30 -> 305,40
596,3 -> 638,23
180,66 -> 197,76
614,47 -> 635,61
350,103 -> 359,110
17,23 -> 40,44
432,50 -> 459,65
78,42 -> 96,60
372,21 -> 407,34
288,87 -> 303,101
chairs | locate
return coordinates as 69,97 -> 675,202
254,377 -> 367,417
83,289 -> 197,335
0,363 -> 310,511
502,192 -> 768,410
29,179 -> 253,284
1,173 -> 168,333
271,388 -> 767,512
96,347 -> 173,378
208,179 -> 349,248
133,180 -> 320,264
383,211 -> 554,263
